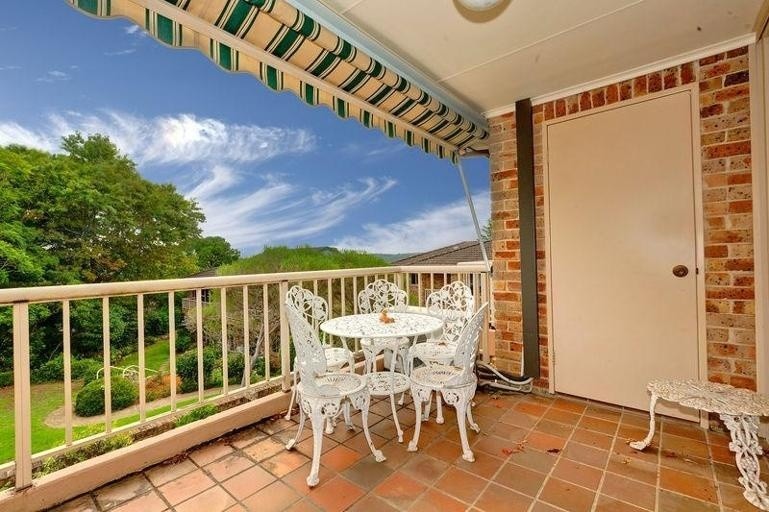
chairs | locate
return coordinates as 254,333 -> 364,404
283,300 -> 387,488
407,301 -> 488,463
283,279 -> 476,444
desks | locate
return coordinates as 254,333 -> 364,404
320,312 -> 443,442
628,376 -> 769,510
